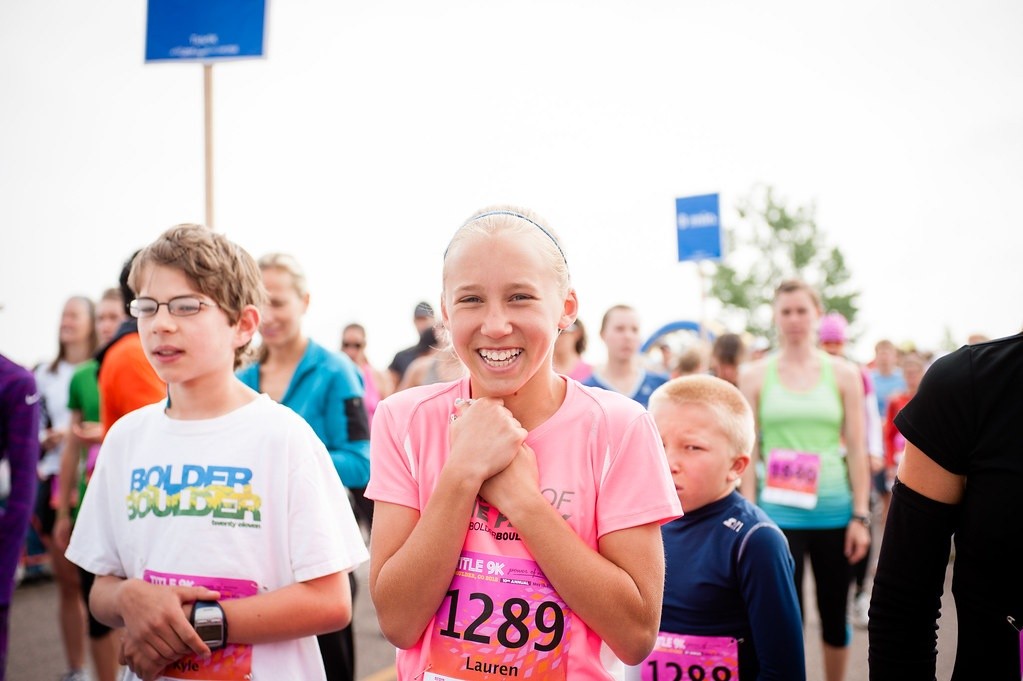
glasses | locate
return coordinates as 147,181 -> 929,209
129,297 -> 219,318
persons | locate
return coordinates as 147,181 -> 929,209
867,331 -> 1023,681
54,288 -> 136,681
0,354 -> 39,681
63,224 -> 372,681
31,295 -> 100,681
738,280 -> 870,681
968,335 -> 990,346
817,312 -> 884,631
341,324 -> 382,434
868,340 -> 952,577
552,318 -> 597,383
234,253 -> 370,680
397,322 -> 470,393
94,249 -> 168,444
382,301 -> 439,394
660,334 -> 744,389
623,374 -> 806,681
582,305 -> 668,410
364,205 -> 684,681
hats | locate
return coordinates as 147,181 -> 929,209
413,302 -> 434,317
817,309 -> 848,344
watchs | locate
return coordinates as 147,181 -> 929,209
189,600 -> 227,650
851,513 -> 871,527
